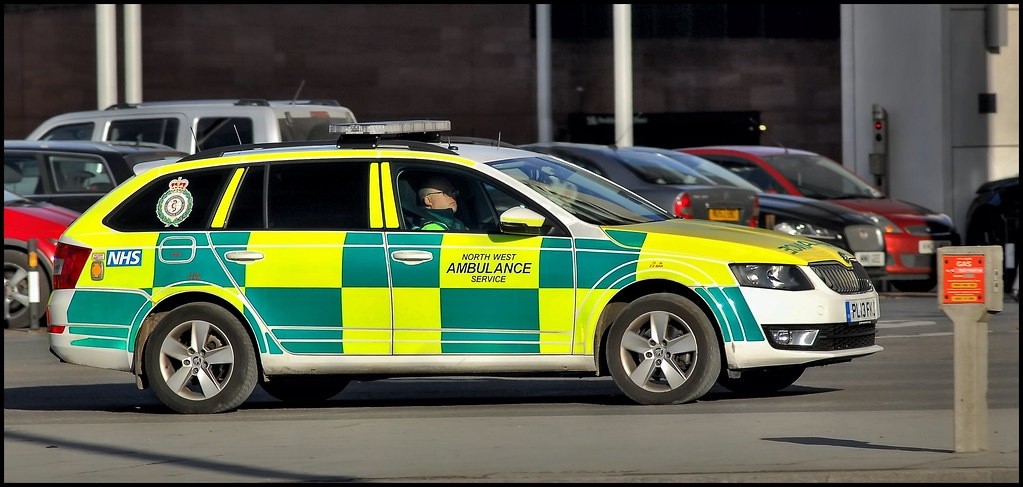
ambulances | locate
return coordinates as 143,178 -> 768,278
47,119 -> 886,412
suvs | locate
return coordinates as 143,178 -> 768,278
2,138 -> 193,214
624,147 -> 886,293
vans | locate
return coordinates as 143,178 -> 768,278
12,98 -> 364,187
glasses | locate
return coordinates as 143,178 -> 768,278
426,188 -> 460,197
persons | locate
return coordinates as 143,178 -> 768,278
413,175 -> 490,233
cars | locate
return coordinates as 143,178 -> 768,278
3,186 -> 84,330
511,141 -> 762,232
666,144 -> 961,296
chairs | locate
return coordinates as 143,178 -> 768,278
4,162 -> 23,193
400,174 -> 423,230
